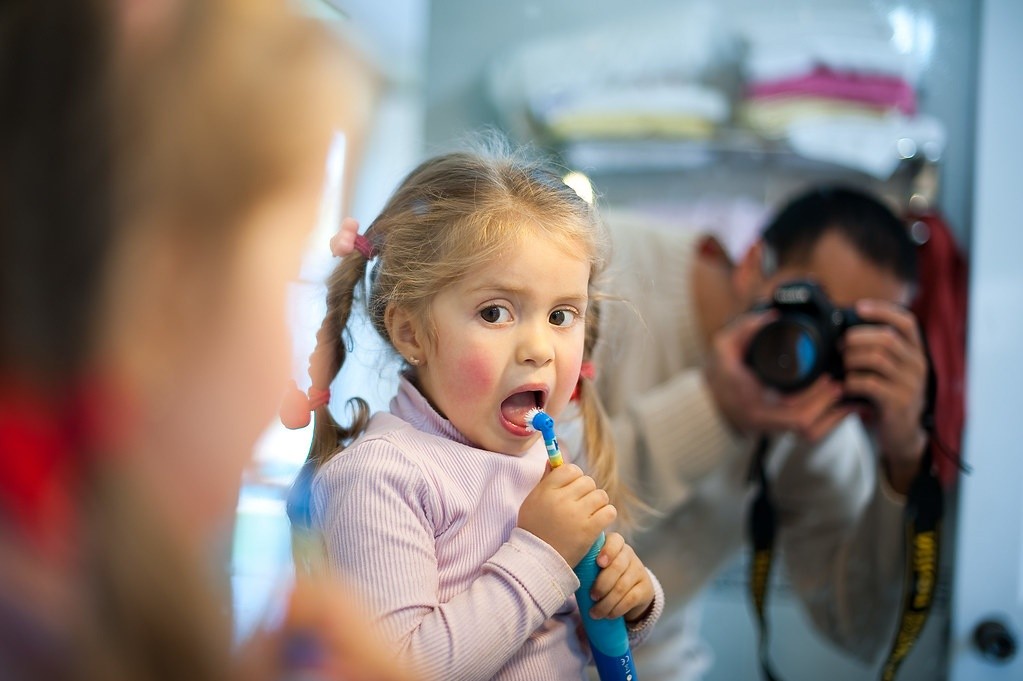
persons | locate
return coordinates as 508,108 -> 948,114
0,0 -> 429,680
552,181 -> 942,681
284,149 -> 666,679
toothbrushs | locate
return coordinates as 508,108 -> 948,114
521,405 -> 639,681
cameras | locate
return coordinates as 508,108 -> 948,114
744,280 -> 881,405
